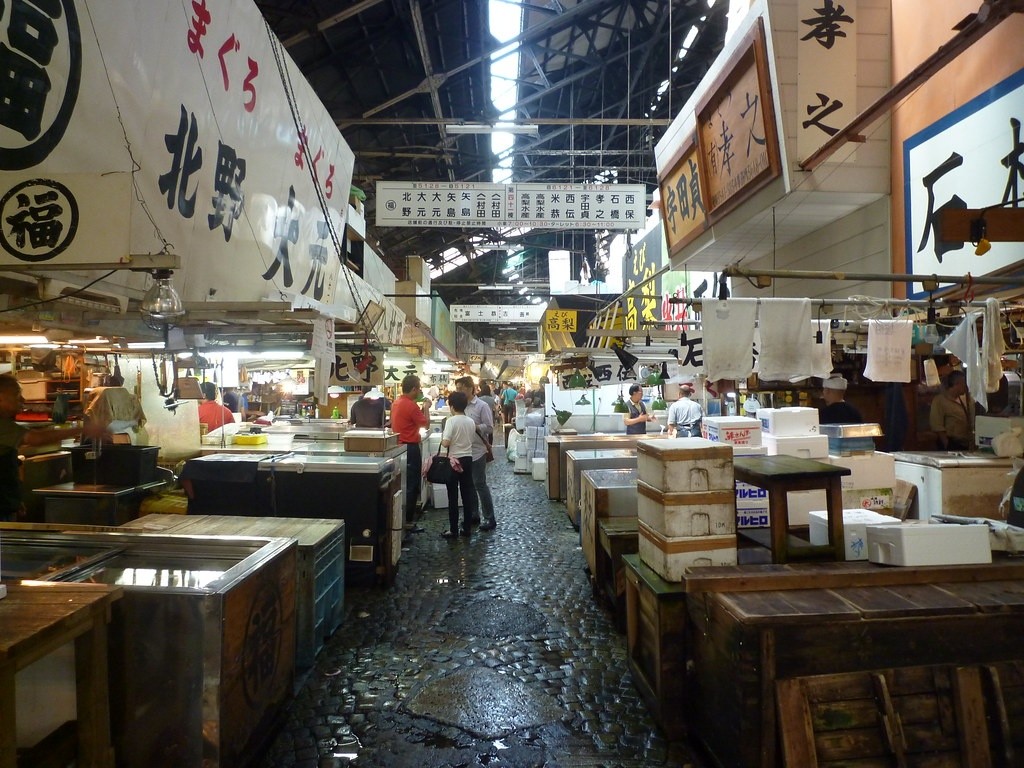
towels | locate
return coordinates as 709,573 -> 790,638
757,297 -> 815,382
982,296 -> 1007,393
701,296 -> 758,384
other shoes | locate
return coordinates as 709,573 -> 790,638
441,530 -> 459,538
477,518 -> 497,530
406,522 -> 426,534
459,528 -> 472,537
461,517 -> 481,526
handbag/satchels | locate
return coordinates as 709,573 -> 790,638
508,400 -> 516,408
484,440 -> 496,462
425,441 -> 454,485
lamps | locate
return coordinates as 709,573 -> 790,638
347,337 -> 376,383
549,342 -> 670,429
131,252 -> 186,331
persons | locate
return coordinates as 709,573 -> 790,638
0,374 -> 114,523
478,380 -> 526,445
454,376 -> 497,530
930,372 -> 970,450
390,375 -> 432,523
350,396 -> 387,430
623,384 -> 657,434
441,392 -> 476,539
667,386 -> 705,438
198,381 -> 234,432
821,378 -> 862,425
222,386 -> 252,421
530,377 -> 550,404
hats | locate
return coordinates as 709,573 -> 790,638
822,374 -> 849,390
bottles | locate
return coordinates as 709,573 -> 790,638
315,406 -> 319,419
302,406 -> 306,416
332,406 -> 339,419
52,393 -> 63,418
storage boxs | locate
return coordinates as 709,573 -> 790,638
0,366 -> 464,768
513,406 -> 1024,768
